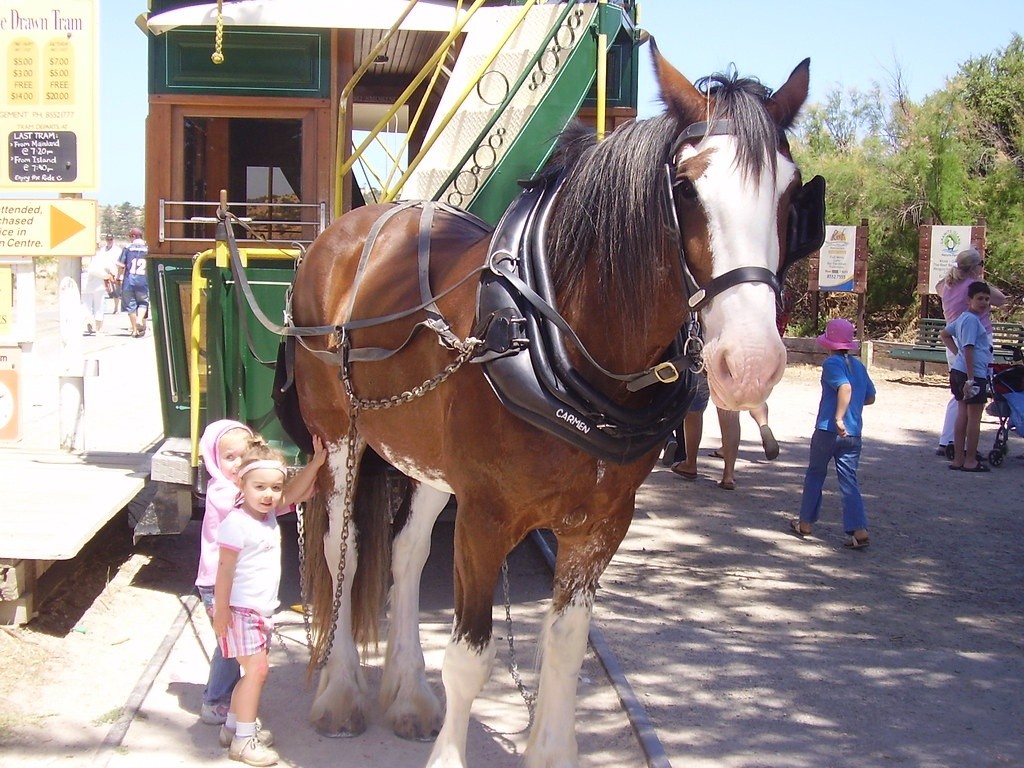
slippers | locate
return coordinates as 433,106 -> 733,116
791,519 -> 813,536
708,450 -> 724,458
843,536 -> 869,549
760,424 -> 782,460
671,461 -> 697,479
717,478 -> 736,490
948,461 -> 964,470
959,459 -> 990,473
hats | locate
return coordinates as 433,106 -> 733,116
817,319 -> 860,351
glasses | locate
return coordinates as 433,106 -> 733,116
977,259 -> 985,267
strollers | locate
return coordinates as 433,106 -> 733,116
987,344 -> 1024,466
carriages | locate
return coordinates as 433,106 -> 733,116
133,0 -> 826,768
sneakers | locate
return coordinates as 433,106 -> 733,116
201,703 -> 230,724
219,722 -> 273,746
228,731 -> 280,767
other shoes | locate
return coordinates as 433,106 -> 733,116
936,446 -> 947,456
662,443 -> 677,464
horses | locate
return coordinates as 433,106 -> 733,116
274,29 -> 810,767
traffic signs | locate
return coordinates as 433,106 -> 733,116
0,198 -> 97,258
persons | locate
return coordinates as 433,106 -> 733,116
670,374 -> 741,489
789,319 -> 876,549
195,419 -> 318,725
663,420 -> 687,471
707,402 -> 779,461
940,282 -> 994,472
936,250 -> 1005,456
81,229 -> 149,337
213,434 -> 328,767
